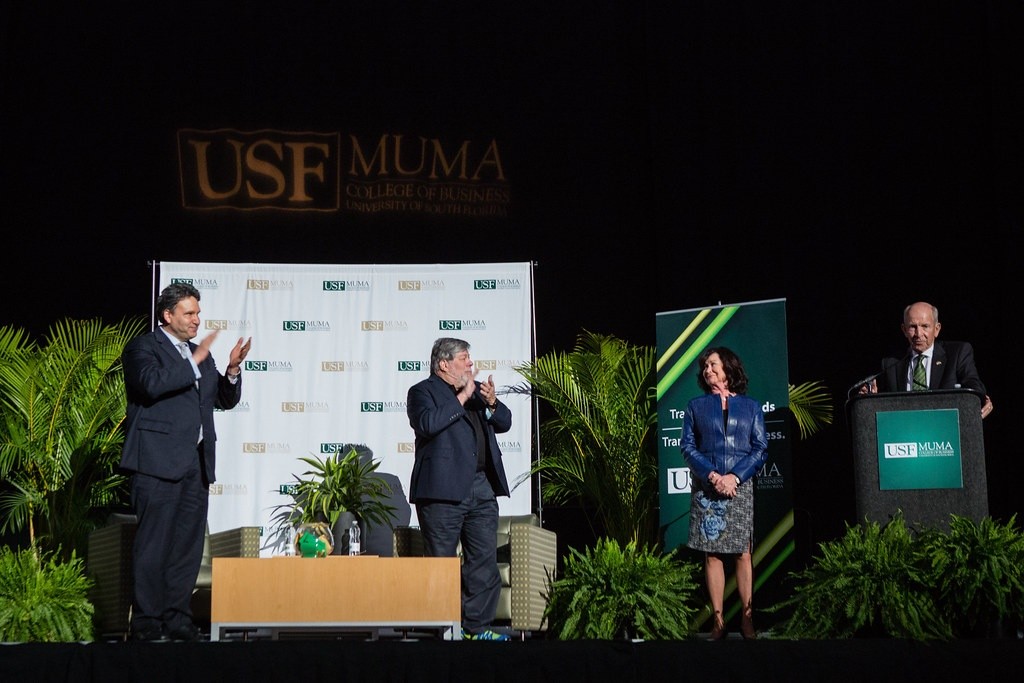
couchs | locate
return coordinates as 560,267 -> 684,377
392,515 -> 558,641
87,502 -> 260,640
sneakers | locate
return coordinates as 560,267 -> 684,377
461,626 -> 509,639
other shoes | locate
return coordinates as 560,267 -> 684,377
704,628 -> 728,642
740,625 -> 756,640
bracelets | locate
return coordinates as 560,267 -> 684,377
709,471 -> 716,480
228,368 -> 241,378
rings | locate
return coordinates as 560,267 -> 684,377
988,406 -> 991,410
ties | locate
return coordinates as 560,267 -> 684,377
178,342 -> 189,359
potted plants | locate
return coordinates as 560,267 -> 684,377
282,448 -> 399,557
544,532 -> 699,641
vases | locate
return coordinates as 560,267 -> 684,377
294,521 -> 334,557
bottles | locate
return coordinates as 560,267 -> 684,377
348,520 -> 360,556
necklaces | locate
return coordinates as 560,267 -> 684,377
721,394 -> 737,401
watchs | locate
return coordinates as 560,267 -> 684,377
489,400 -> 497,409
731,473 -> 740,485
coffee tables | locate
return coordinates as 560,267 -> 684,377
210,557 -> 464,642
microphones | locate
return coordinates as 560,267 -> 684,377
848,348 -> 912,400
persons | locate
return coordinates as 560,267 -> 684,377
859,302 -> 994,420
121,283 -> 252,644
679,346 -> 767,642
407,338 -> 512,641
